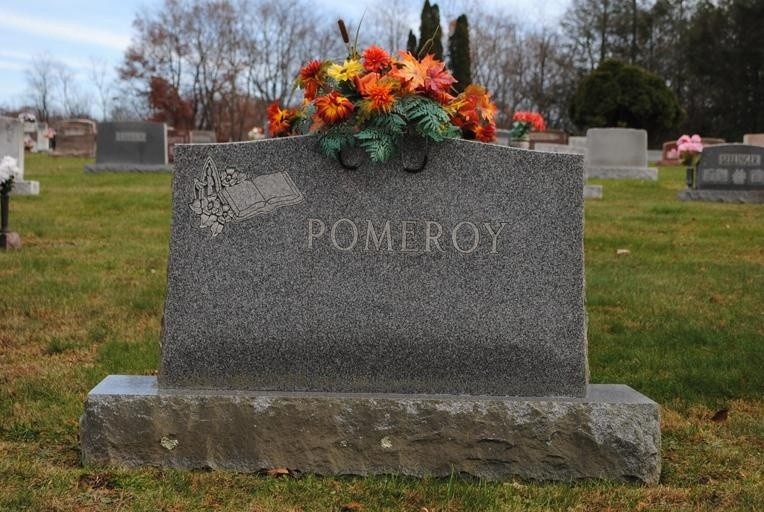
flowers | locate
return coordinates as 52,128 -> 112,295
666,133 -> 704,167
263,6 -> 548,174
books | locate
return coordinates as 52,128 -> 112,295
219,169 -> 304,223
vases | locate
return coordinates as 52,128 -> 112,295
686,167 -> 694,188
